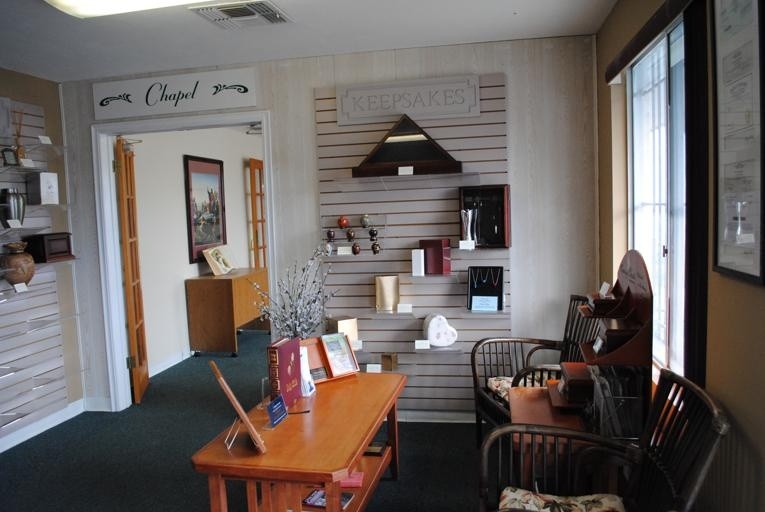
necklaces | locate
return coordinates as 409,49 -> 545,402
471,269 -> 479,288
480,269 -> 489,283
490,268 -> 501,287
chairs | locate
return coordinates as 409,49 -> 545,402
472,293 -> 730,512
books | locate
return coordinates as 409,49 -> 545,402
313,472 -> 365,488
364,440 -> 386,456
303,488 -> 356,511
266,331 -> 361,408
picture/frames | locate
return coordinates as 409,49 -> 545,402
182,153 -> 228,264
708,0 -> 765,288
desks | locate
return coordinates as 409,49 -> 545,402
186,267 -> 270,357
